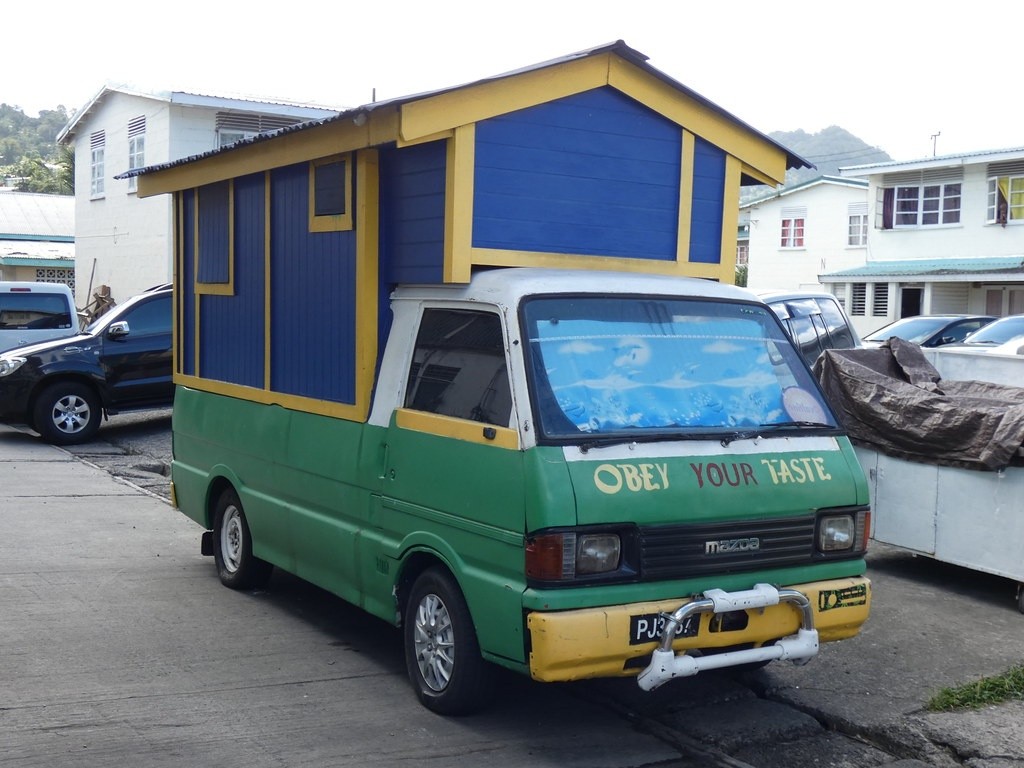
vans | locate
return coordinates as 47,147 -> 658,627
0,281 -> 80,356
750,291 -> 862,376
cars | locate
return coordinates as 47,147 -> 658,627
861,312 -> 999,348
936,314 -> 1024,355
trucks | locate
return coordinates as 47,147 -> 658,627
114,37 -> 872,718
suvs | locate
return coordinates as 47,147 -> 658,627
0,282 -> 176,445
